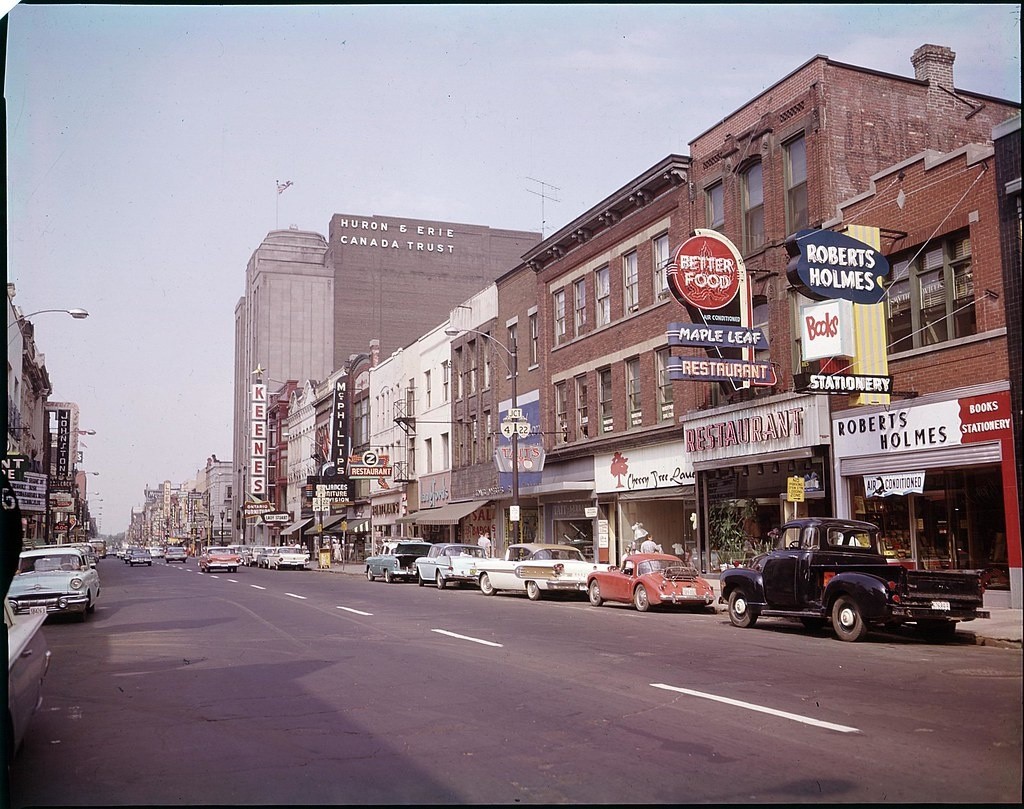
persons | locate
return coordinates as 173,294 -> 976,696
58,555 -> 74,570
332,539 -> 342,566
641,534 -> 664,554
620,547 -> 641,568
289,541 -> 307,550
460,530 -> 491,558
684,549 -> 696,572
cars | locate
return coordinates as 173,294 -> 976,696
198,546 -> 243,572
587,554 -> 716,611
476,543 -> 612,600
35,541 -> 106,570
226,545 -> 310,570
106,545 -> 165,567
4,601 -> 51,764
6,548 -> 101,621
364,538 -> 434,583
415,543 -> 501,590
165,547 -> 188,563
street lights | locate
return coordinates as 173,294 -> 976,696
283,433 -> 323,569
220,509 -> 225,546
209,512 -> 214,546
68,471 -> 99,540
46,429 -> 96,543
445,326 -> 520,561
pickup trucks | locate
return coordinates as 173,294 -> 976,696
718,518 -> 990,641
